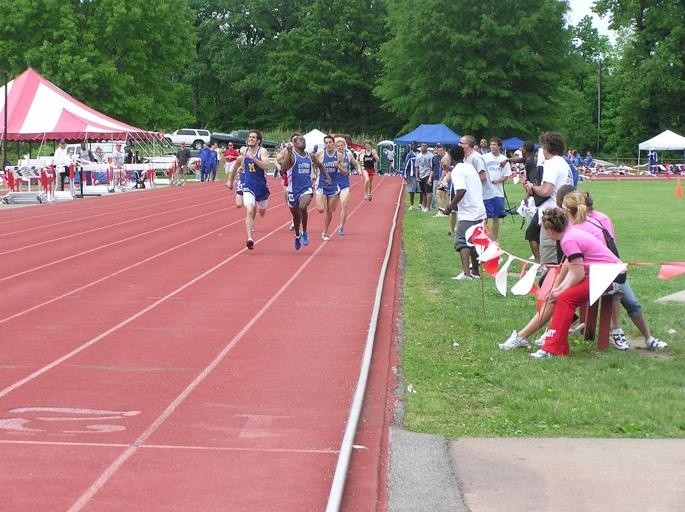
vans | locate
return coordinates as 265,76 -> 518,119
60,141 -> 132,164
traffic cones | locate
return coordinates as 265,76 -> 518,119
673,175 -> 685,200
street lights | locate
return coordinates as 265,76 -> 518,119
594,52 -> 605,157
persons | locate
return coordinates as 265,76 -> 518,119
54,140 -> 144,192
223,131 -> 269,248
274,132 -> 396,250
404,133 -> 668,357
177,140 -> 221,186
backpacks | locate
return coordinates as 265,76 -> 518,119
584,217 -> 619,258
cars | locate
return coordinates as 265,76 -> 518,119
330,133 -> 368,152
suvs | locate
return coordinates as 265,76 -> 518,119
156,128 -> 211,150
584,158 -> 618,175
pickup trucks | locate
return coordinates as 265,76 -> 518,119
206,130 -> 282,153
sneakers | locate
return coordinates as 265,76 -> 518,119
498,314 -> 586,360
294,231 -> 309,250
645,337 -> 668,350
322,233 -> 332,241
609,328 -> 629,350
339,228 -> 344,235
450,261 -> 481,280
